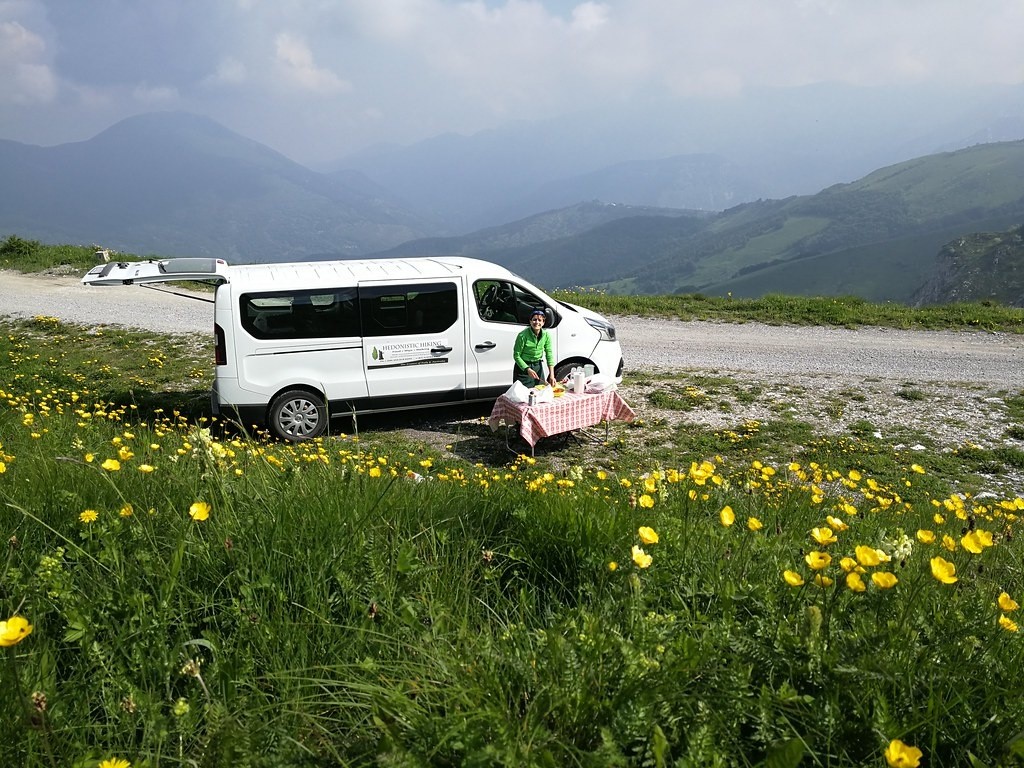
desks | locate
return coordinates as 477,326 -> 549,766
488,378 -> 635,458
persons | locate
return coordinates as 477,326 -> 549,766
513,311 -> 556,388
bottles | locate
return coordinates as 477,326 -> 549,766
577,367 -> 582,374
528,391 -> 536,406
571,368 -> 576,380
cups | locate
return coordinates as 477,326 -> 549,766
585,365 -> 594,377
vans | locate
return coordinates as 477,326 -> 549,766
81,256 -> 625,445
